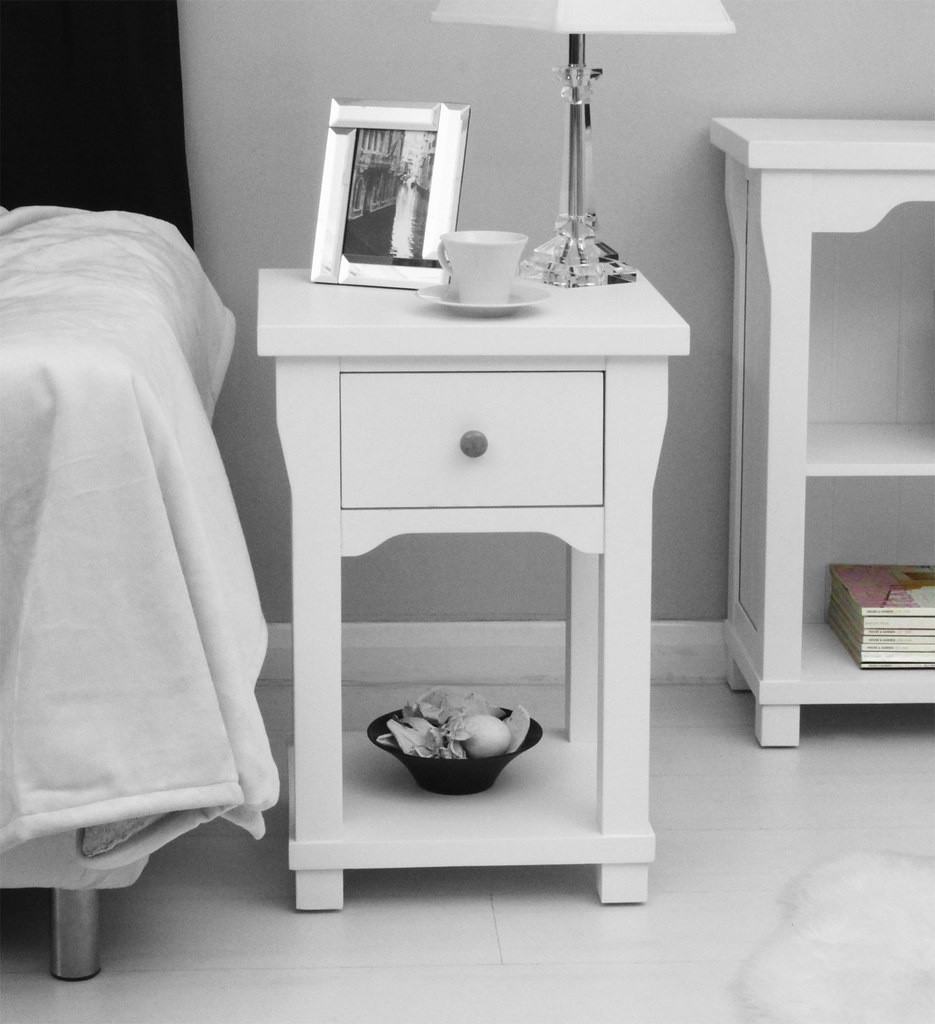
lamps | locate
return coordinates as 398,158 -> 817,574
431,0 -> 735,288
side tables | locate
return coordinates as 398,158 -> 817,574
249,264 -> 692,913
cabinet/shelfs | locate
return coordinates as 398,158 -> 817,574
708,119 -> 935,747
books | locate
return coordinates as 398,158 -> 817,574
825,563 -> 935,671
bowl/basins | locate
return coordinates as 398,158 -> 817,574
367,707 -> 544,796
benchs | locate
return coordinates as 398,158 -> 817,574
0,0 -> 282,983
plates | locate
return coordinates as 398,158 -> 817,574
417,282 -> 554,316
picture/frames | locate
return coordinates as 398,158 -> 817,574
312,96 -> 473,291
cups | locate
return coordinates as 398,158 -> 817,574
437,230 -> 529,303
